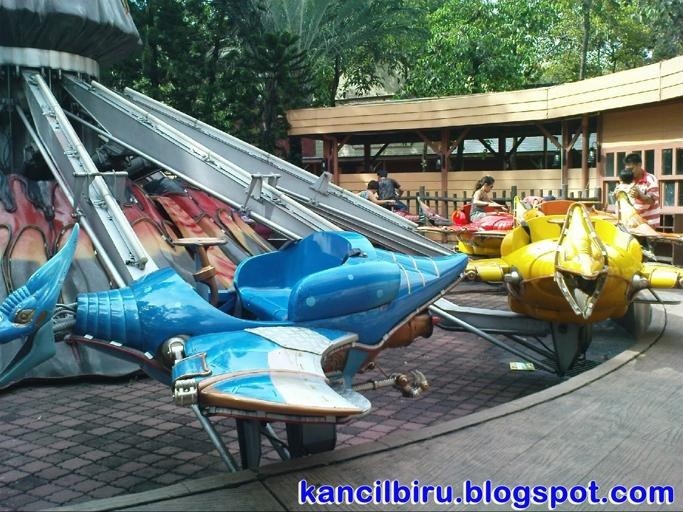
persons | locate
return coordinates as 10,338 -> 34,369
373,171 -> 407,209
613,153 -> 661,250
612,169 -> 653,225
524,195 -> 555,209
470,176 -> 508,223
356,180 -> 395,206
